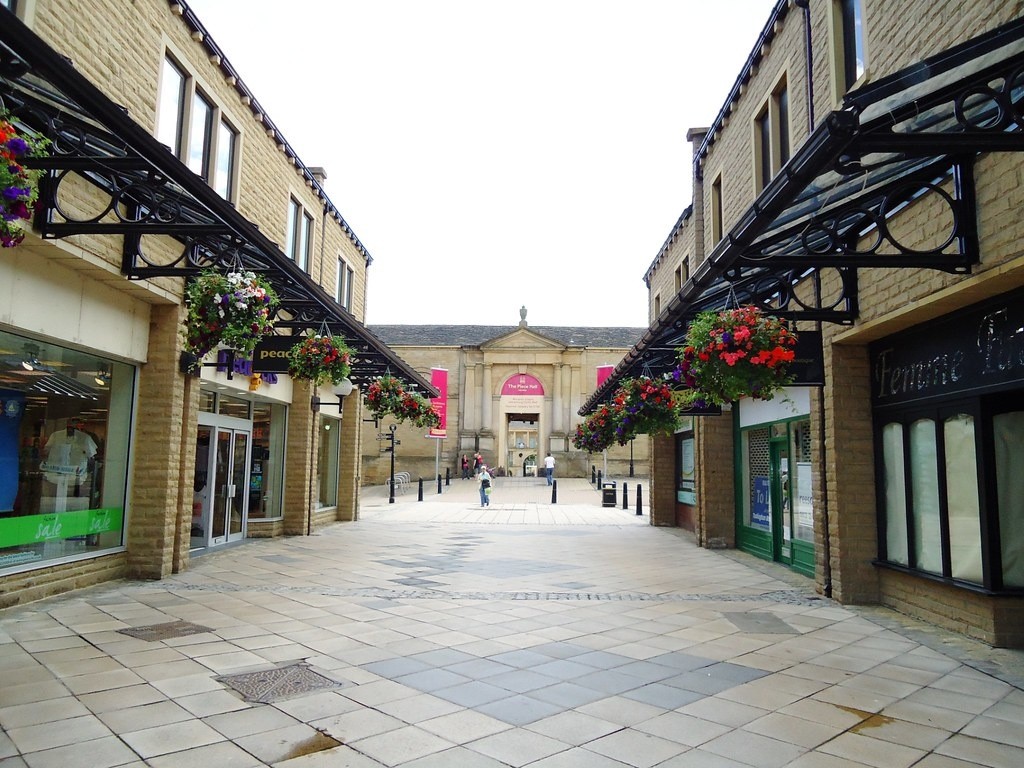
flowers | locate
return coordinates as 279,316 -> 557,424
365,374 -> 446,431
0,107 -> 49,250
572,373 -> 681,456
674,304 -> 798,408
178,267 -> 272,367
288,330 -> 356,392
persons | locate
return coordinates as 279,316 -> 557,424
544,453 -> 555,486
472,453 -> 495,480
477,466 -> 491,507
462,454 -> 471,480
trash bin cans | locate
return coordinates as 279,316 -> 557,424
601,482 -> 617,507
